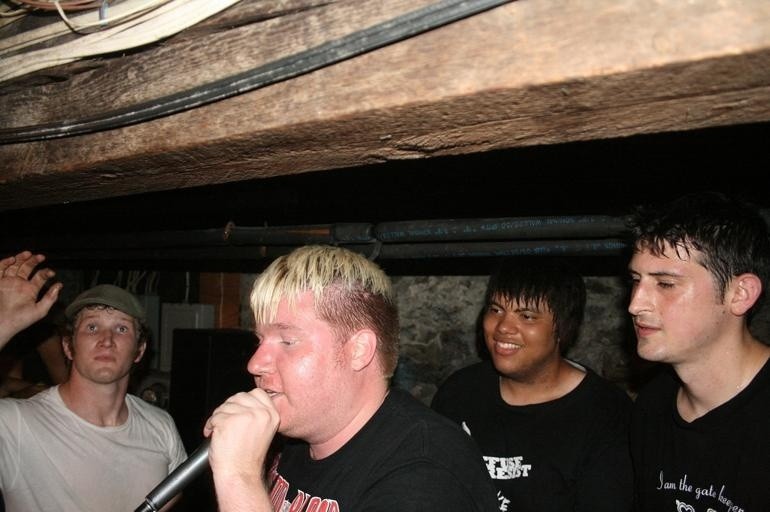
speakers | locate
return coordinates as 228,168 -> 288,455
169,328 -> 259,438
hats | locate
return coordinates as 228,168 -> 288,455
64,283 -> 146,319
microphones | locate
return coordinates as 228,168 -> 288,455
134,430 -> 212,512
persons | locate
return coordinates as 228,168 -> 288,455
0,250 -> 191,512
430,251 -> 635,512
203,245 -> 501,512
622,188 -> 770,512
